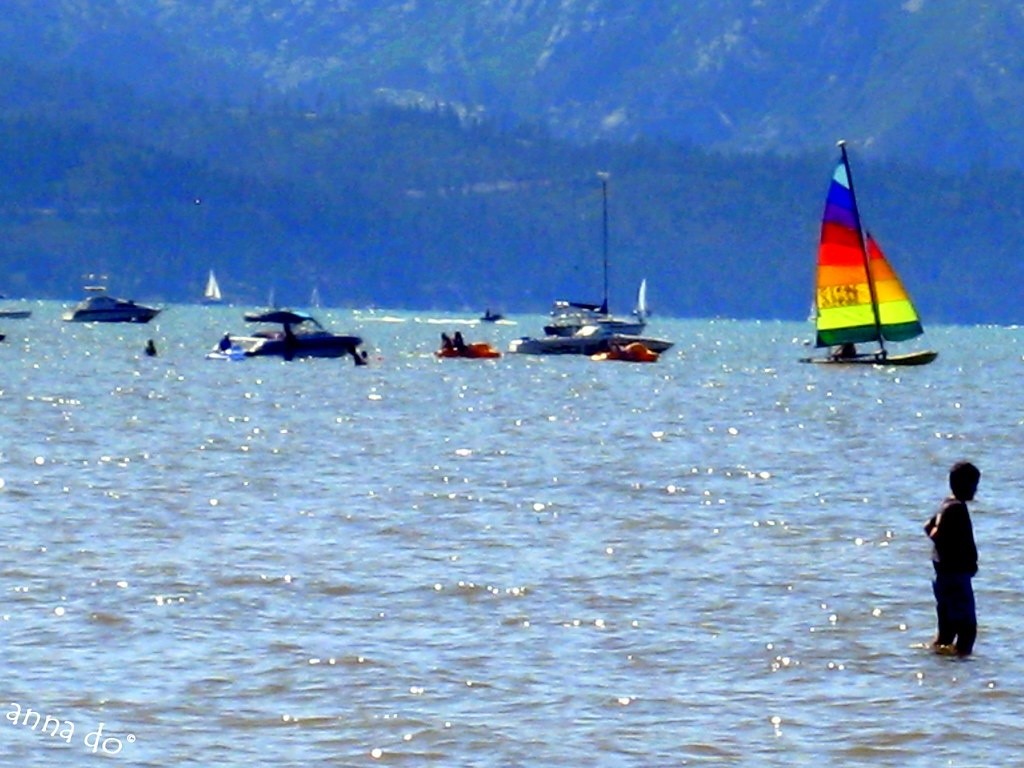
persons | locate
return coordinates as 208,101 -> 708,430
486,309 -> 489,318
835,343 -> 856,360
220,333 -> 231,351
925,462 -> 980,656
442,332 -> 463,348
146,339 -> 156,356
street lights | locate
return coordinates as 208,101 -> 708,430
63,295 -> 163,324
205,312 -> 363,360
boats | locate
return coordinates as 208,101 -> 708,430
508,326 -> 676,361
439,343 -> 499,358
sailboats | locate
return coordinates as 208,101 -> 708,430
800,139 -> 937,366
544,179 -> 646,337
